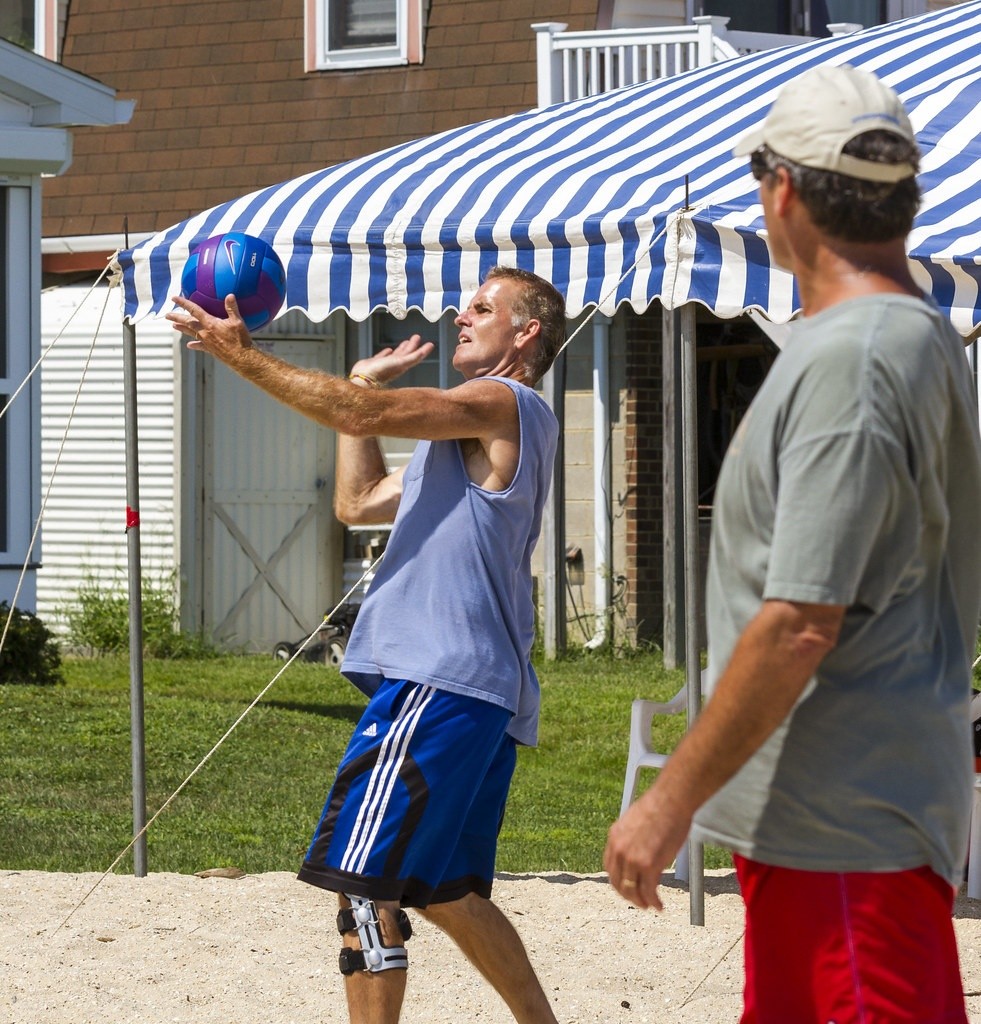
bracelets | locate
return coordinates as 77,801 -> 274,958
346,374 -> 380,389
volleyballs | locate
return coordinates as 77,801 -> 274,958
182,231 -> 288,333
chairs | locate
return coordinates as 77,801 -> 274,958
966,692 -> 981,903
618,668 -> 707,883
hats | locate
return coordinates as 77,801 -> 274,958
732,67 -> 915,184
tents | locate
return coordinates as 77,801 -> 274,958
103,0 -> 980,876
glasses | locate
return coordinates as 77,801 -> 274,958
750,142 -> 776,180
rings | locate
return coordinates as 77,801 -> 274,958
623,879 -> 638,890
194,332 -> 198,340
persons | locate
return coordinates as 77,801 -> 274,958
163,268 -> 565,1024
599,62 -> 981,1024
